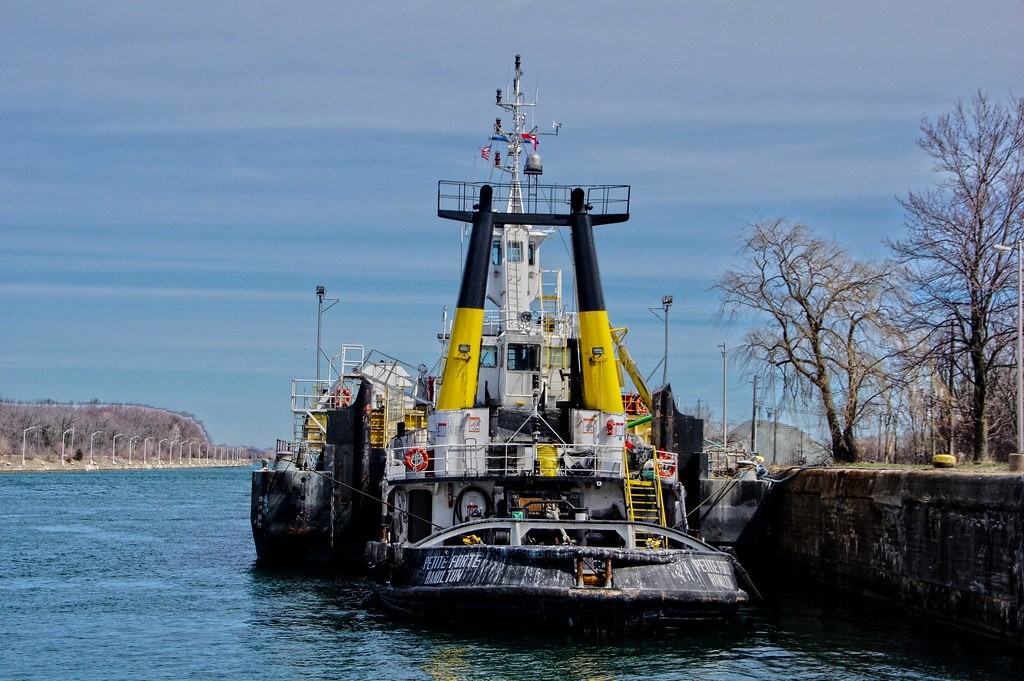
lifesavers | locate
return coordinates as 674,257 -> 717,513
658,455 -> 676,477
333,387 -> 351,407
405,448 -> 428,471
636,397 -> 649,413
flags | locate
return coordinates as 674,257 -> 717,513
480,145 -> 489,160
530,136 -> 536,151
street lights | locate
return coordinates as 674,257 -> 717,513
188,441 -> 197,464
61,429 -> 75,466
158,439 -> 168,465
144,437 -> 154,464
112,433 -> 124,464
90,431 -> 102,464
992,241 -> 1023,453
22,427 -> 37,466
170,440 -> 179,464
180,441 -> 188,464
199,442 -> 206,463
129,435 -> 140,464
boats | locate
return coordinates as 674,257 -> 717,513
249,53 -> 750,625
